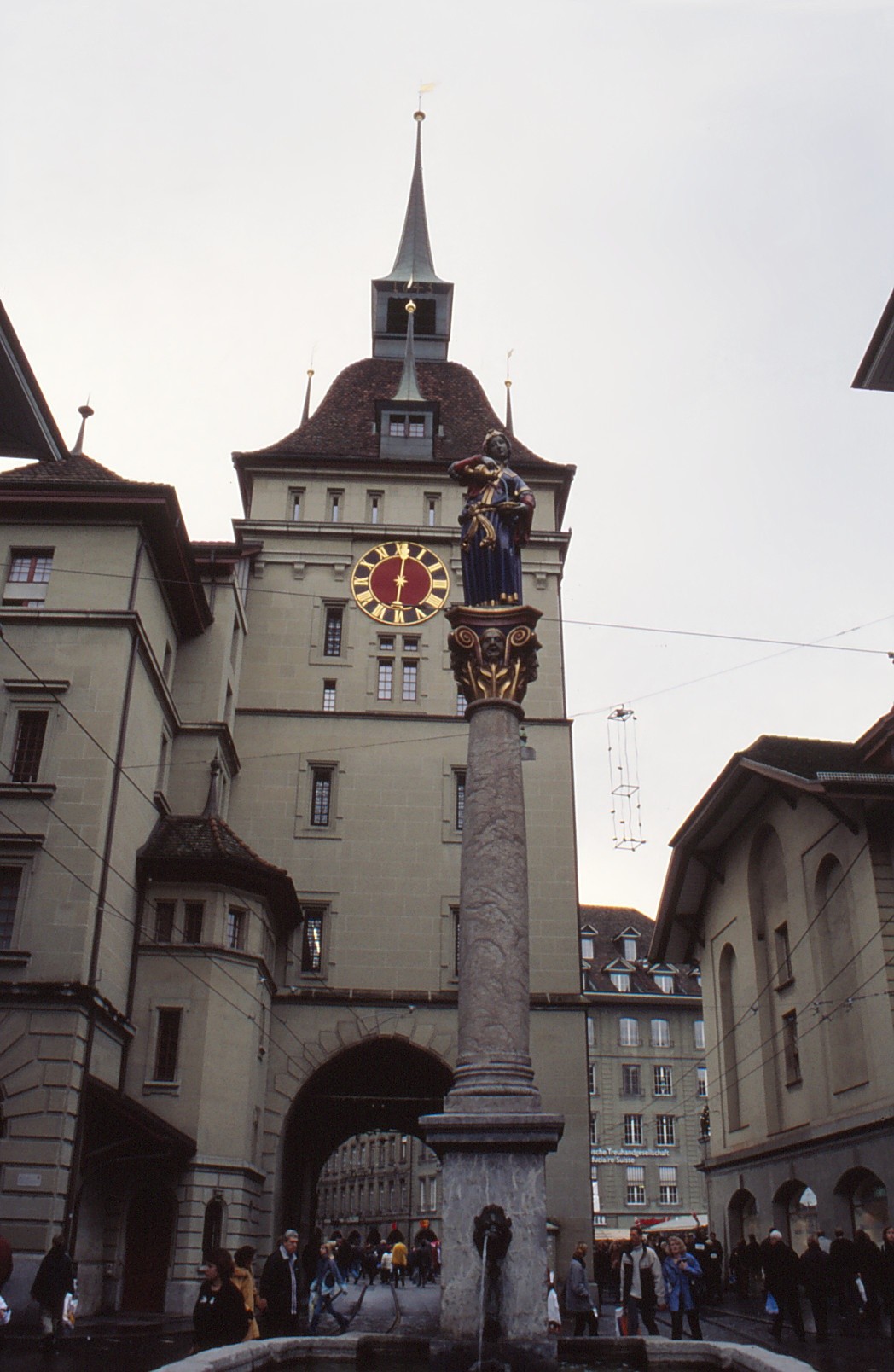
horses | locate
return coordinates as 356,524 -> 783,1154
322,1236 -> 438,1289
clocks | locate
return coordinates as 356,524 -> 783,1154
350,540 -> 453,624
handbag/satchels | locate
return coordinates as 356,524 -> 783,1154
62,1292 -> 79,1331
615,1303 -> 642,1337
308,1291 -> 314,1323
765,1292 -> 779,1315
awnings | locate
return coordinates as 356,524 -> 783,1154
593,1228 -> 631,1240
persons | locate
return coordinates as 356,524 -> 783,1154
190,1247 -> 249,1358
620,1227 -> 666,1336
759,1227 -> 805,1341
230,1246 -> 268,1342
306,1242 -> 350,1335
799,1228 -> 893,1348
565,1241 -> 600,1336
730,1233 -> 762,1309
594,1229 -> 723,1309
479,627 -> 505,661
662,1237 -> 704,1341
0,1237 -> 15,1290
260,1229 -> 302,1339
30,1233 -> 75,1352
329,1238 -> 441,1289
448,428 -> 536,607
